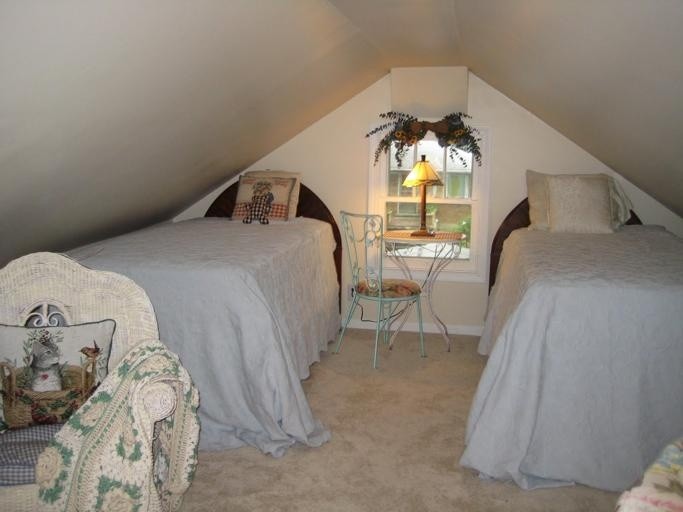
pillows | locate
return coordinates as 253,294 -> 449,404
527,169 -> 627,238
230,177 -> 296,223
546,178 -> 612,239
245,171 -> 302,225
0,321 -> 115,428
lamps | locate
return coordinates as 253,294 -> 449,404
403,153 -> 444,240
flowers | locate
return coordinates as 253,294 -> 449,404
367,105 -> 488,171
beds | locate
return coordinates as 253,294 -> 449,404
57,170 -> 339,450
490,191 -> 682,471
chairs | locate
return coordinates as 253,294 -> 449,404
333,208 -> 427,369
1,250 -> 203,512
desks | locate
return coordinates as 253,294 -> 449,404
371,229 -> 468,358
